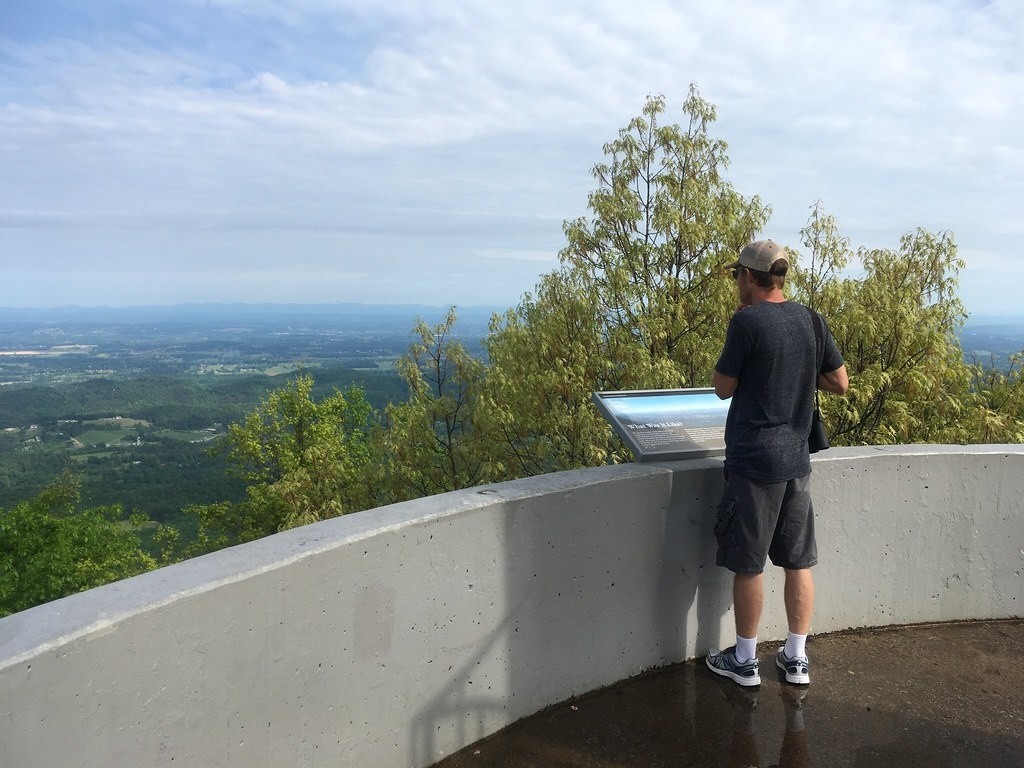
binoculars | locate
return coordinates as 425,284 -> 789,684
808,409 -> 830,453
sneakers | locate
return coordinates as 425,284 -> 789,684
774,647 -> 811,684
705,643 -> 760,686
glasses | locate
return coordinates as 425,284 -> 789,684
731,268 -> 757,280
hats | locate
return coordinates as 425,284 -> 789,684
723,239 -> 790,276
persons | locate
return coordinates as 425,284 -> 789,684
706,238 -> 849,687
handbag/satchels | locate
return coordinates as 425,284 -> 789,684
809,409 -> 829,454
